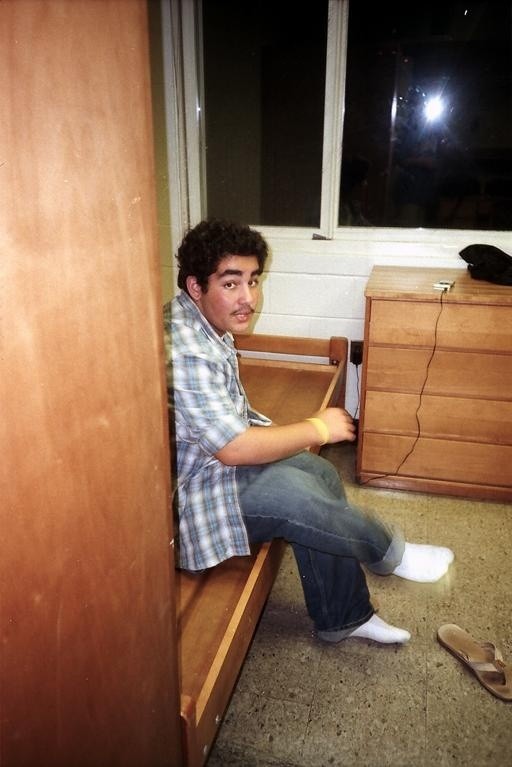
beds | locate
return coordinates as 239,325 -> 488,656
167,332 -> 347,766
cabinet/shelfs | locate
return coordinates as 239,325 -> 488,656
356,265 -> 512,504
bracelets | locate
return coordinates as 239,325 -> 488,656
305,417 -> 328,446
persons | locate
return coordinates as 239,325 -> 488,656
160,217 -> 455,647
391,60 -> 481,230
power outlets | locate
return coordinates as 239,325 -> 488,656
350,340 -> 363,363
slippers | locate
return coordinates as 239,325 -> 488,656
435,623 -> 511,700
